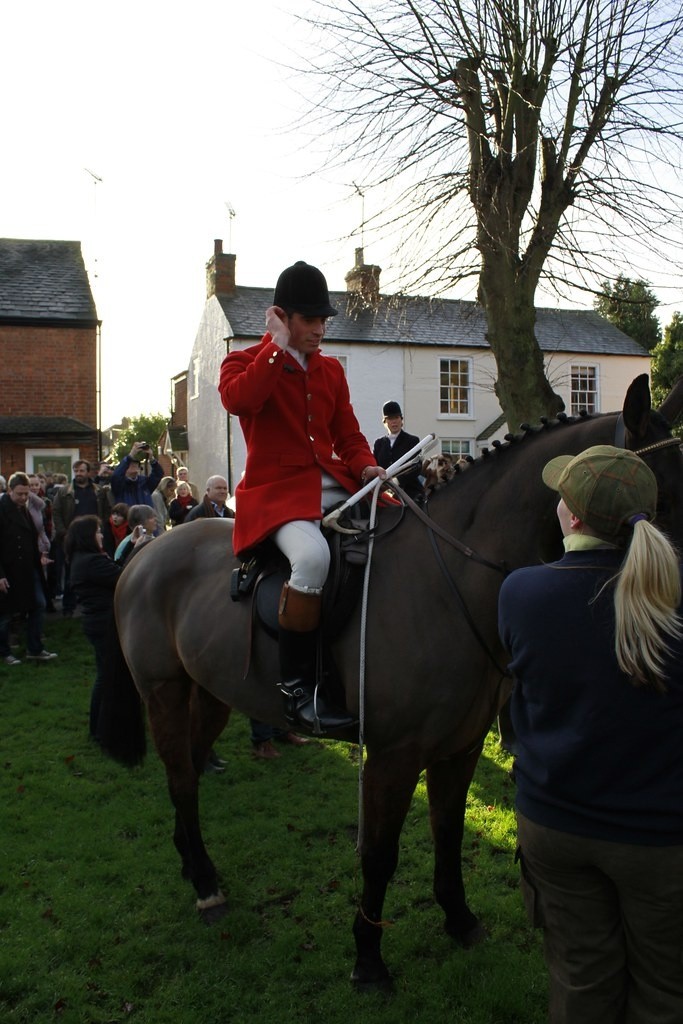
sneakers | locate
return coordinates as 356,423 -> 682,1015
26,649 -> 57,660
4,654 -> 21,665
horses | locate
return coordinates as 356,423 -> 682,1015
112,373 -> 680,984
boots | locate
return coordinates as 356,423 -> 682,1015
277,581 -> 359,731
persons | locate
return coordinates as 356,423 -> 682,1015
217,260 -> 386,736
184,475 -> 235,525
0,442 -> 199,665
65,516 -> 154,768
498,445 -> 683,1024
203,717 -> 312,771
374,401 -> 424,501
421,452 -> 452,487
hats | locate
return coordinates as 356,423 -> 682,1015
542,445 -> 658,535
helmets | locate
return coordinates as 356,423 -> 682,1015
274,261 -> 338,318
383,401 -> 402,417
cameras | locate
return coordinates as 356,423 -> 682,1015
135,526 -> 143,539
137,441 -> 149,459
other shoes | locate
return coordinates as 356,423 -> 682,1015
204,751 -> 229,772
46,601 -> 56,612
251,742 -> 283,758
40,632 -> 47,642
8,633 -> 20,648
275,733 -> 310,746
63,611 -> 72,619
